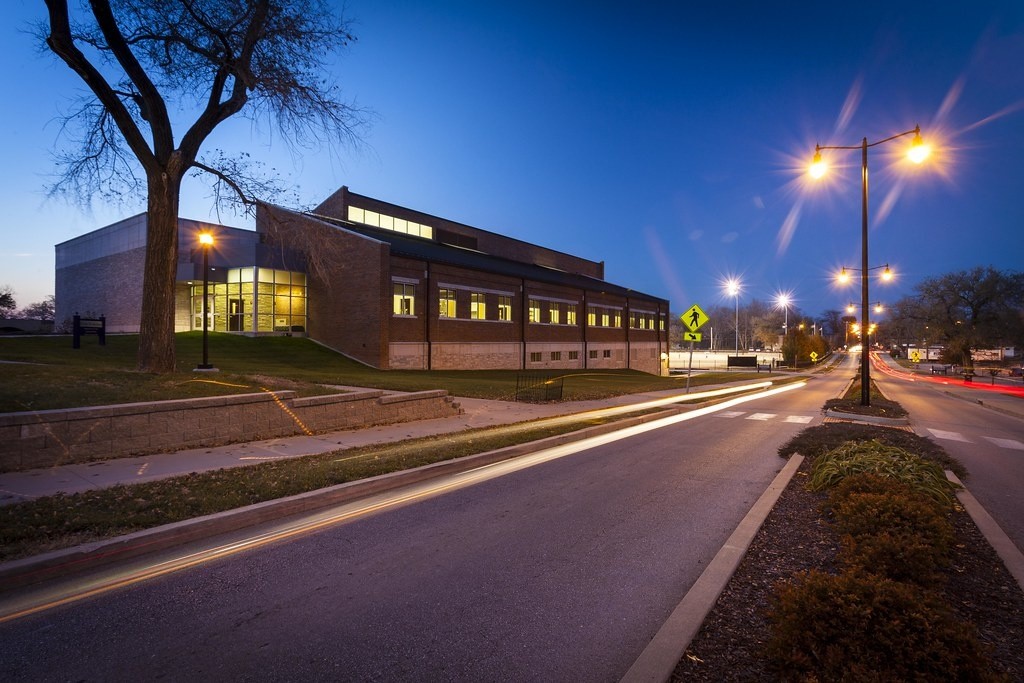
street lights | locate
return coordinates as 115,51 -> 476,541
810,124 -> 931,405
729,281 -> 740,356
780,295 -> 788,335
193,232 -> 220,371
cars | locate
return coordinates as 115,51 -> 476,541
1009,368 -> 1024,376
748,347 -> 761,352
